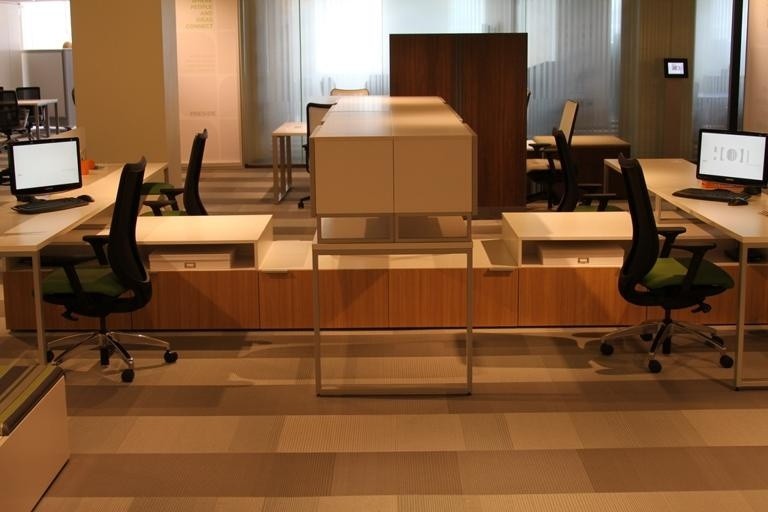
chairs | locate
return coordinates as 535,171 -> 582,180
331,87 -> 370,98
526,99 -> 579,207
299,103 -> 335,209
45,157 -> 177,383
16,87 -> 40,99
143,129 -> 208,214
546,128 -> 620,210
599,151 -> 737,377
0,90 -> 30,141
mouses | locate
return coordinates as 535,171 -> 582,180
77,194 -> 94,203
729,198 -> 748,205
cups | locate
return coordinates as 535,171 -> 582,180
80,160 -> 89,175
89,160 -> 95,169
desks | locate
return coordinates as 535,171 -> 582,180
533,130 -> 631,210
17,99 -> 58,141
272,121 -> 310,203
603,157 -> 768,512
1,161 -> 169,363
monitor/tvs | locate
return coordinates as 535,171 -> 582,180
696,129 -> 768,194
7,137 -> 82,202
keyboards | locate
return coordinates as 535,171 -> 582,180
672,188 -> 750,201
11,197 -> 88,214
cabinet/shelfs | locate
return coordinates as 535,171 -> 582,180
389,33 -> 527,218
